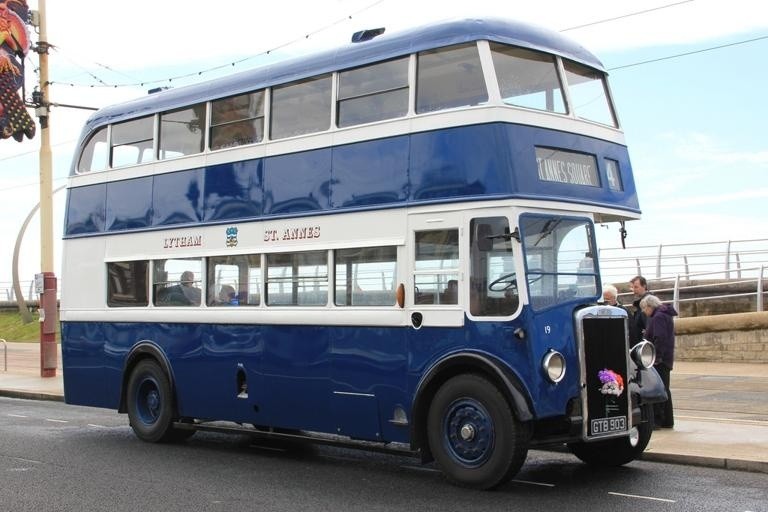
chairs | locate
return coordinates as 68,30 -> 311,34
108,268 -> 134,301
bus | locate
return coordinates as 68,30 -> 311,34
59,15 -> 676,490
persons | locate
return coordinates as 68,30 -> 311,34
601,276 -> 678,430
218,285 -> 236,301
180,271 -> 194,288
